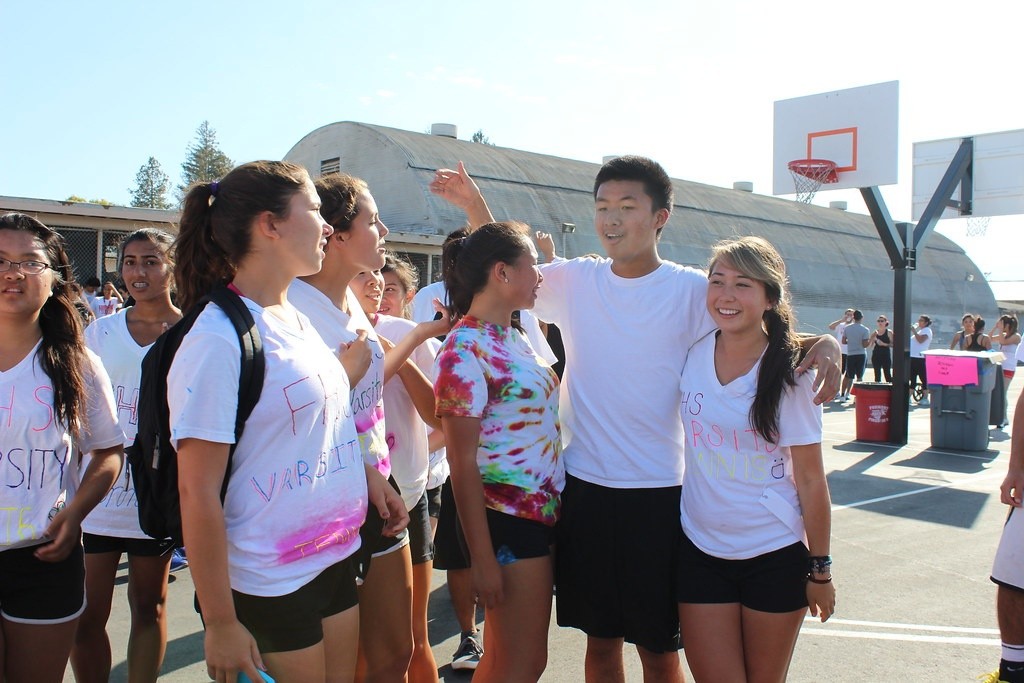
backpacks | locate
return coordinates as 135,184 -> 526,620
122,285 -> 266,545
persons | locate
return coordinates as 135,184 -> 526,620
950,314 -> 975,350
425,222 -> 565,683
676,236 -> 836,683
407,229 -> 559,670
979,386 -> 1024,683
829,309 -> 855,400
840,310 -> 871,404
168,161 -> 411,683
0,215 -> 126,683
1015,332 -> 1024,366
962,317 -> 991,351
69,277 -> 137,328
69,227 -> 185,683
909,315 -> 932,405
869,315 -> 894,382
988,314 -> 1022,428
285,174 -> 451,683
430,156 -> 842,683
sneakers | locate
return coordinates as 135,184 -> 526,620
918,397 -> 929,405
451,627 -> 484,669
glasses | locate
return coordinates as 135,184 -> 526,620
0,258 -> 55,275
878,320 -> 884,322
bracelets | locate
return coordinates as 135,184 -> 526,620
805,569 -> 832,584
806,555 -> 832,579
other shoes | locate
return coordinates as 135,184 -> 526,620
169,549 -> 189,573
835,392 -> 840,398
846,392 -> 850,400
839,396 -> 846,402
997,419 -> 1009,428
177,546 -> 187,560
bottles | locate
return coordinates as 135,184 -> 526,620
847,318 -> 851,321
914,323 -> 919,329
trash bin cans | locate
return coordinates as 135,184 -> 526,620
920,349 -> 1007,451
850,383 -> 893,442
